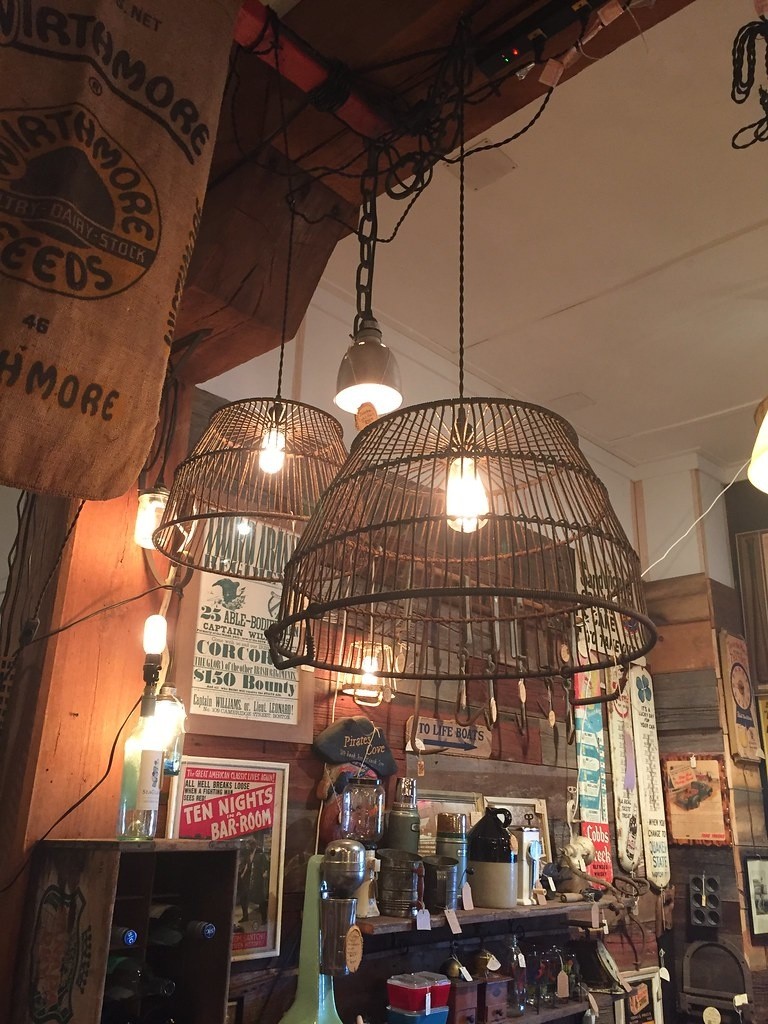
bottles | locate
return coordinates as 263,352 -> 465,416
501,935 -> 579,1018
116,697 -> 162,841
103,904 -> 217,1024
155,687 -> 186,776
340,777 -> 385,844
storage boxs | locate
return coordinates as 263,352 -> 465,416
387,972 -> 515,1024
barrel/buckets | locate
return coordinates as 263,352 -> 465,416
377,849 -> 424,918
423,856 -> 459,915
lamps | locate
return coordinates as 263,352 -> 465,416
339,555 -> 399,707
133,371 -> 174,552
266,76 -> 655,682
334,134 -> 404,426
140,614 -> 168,716
150,177 -> 377,583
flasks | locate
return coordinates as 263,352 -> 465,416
435,813 -> 467,909
386,777 -> 421,854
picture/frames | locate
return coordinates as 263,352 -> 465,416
483,795 -> 552,866
660,750 -> 736,848
417,790 -> 484,856
168,755 -> 289,963
743,855 -> 768,937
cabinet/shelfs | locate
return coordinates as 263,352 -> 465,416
27,836 -> 247,1024
319,900 -> 617,1024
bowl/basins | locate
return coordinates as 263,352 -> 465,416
386,971 -> 451,1024
